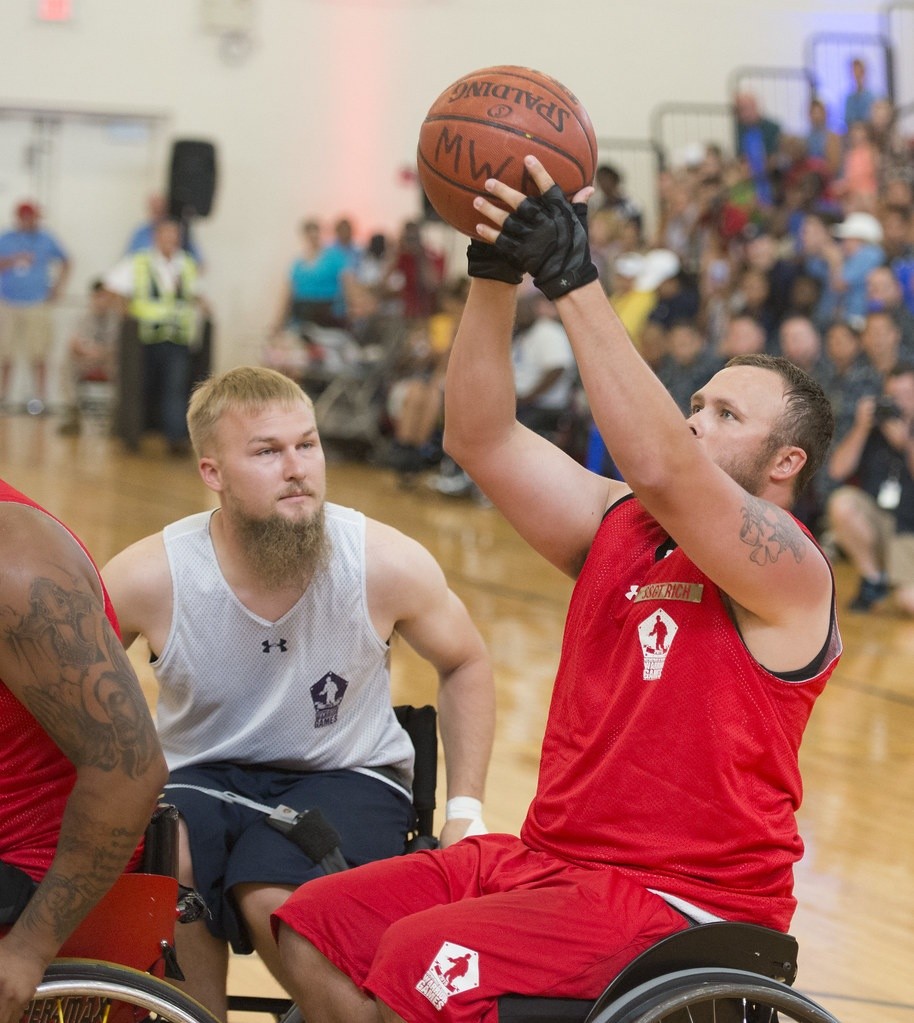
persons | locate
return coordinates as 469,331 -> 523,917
99,363 -> 498,1023
269,153 -> 844,1023
0,203 -> 68,411
2,479 -> 171,1023
88,193 -> 209,460
267,60 -> 914,614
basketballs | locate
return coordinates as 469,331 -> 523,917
417,62 -> 598,246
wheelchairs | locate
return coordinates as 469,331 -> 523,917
19,704 -> 838,1023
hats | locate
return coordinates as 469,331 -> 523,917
18,205 -> 41,220
631,249 -> 680,291
831,211 -> 884,245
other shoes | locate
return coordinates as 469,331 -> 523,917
847,570 -> 897,611
167,440 -> 195,459
125,433 -> 144,456
